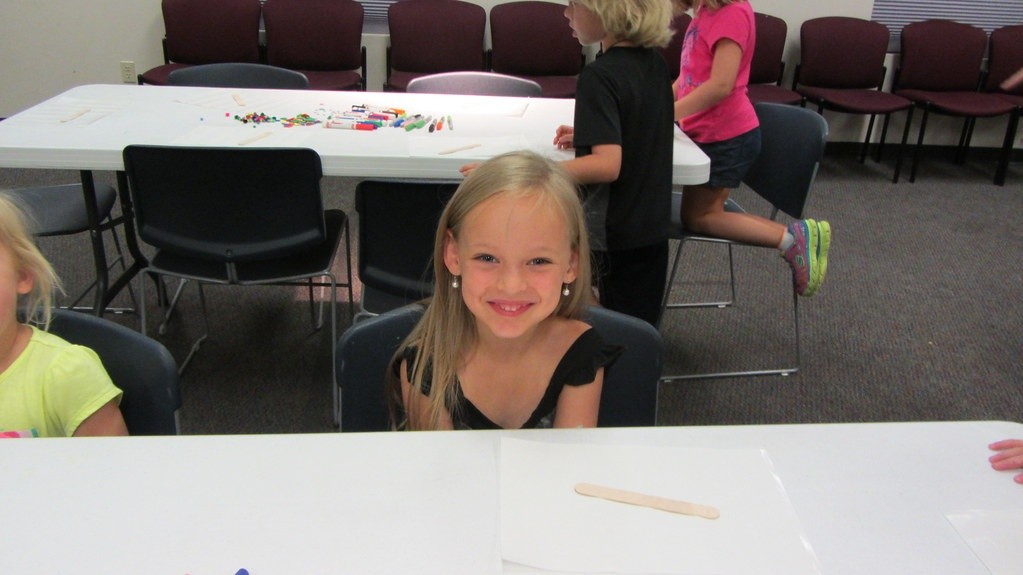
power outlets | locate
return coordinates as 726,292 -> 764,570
121,61 -> 137,83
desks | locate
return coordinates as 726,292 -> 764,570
0,85 -> 711,184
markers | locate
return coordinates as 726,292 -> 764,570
428,118 -> 437,132
437,115 -> 445,130
447,116 -> 453,130
323,104 -> 432,132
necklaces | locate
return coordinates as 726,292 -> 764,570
610,39 -> 626,47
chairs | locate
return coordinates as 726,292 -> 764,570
663,102 -> 829,379
384,2 -> 584,97
336,181 -> 663,432
20,307 -> 181,435
139,1 -> 366,433
745,13 -> 1023,185
0,183 -> 137,310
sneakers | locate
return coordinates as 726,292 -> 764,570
779,218 -> 832,297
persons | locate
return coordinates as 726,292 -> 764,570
672,0 -> 831,298
385,148 -> 623,432
0,196 -> 129,439
459,0 -> 681,326
988,439 -> 1023,484
